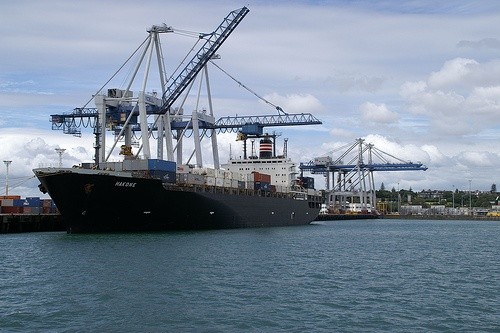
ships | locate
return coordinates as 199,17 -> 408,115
32,129 -> 327,233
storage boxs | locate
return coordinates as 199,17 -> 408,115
0,195 -> 60,214
82,158 -> 282,192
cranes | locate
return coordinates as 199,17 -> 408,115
113,112 -> 323,189
49,6 -> 250,159
299,138 -> 428,213
